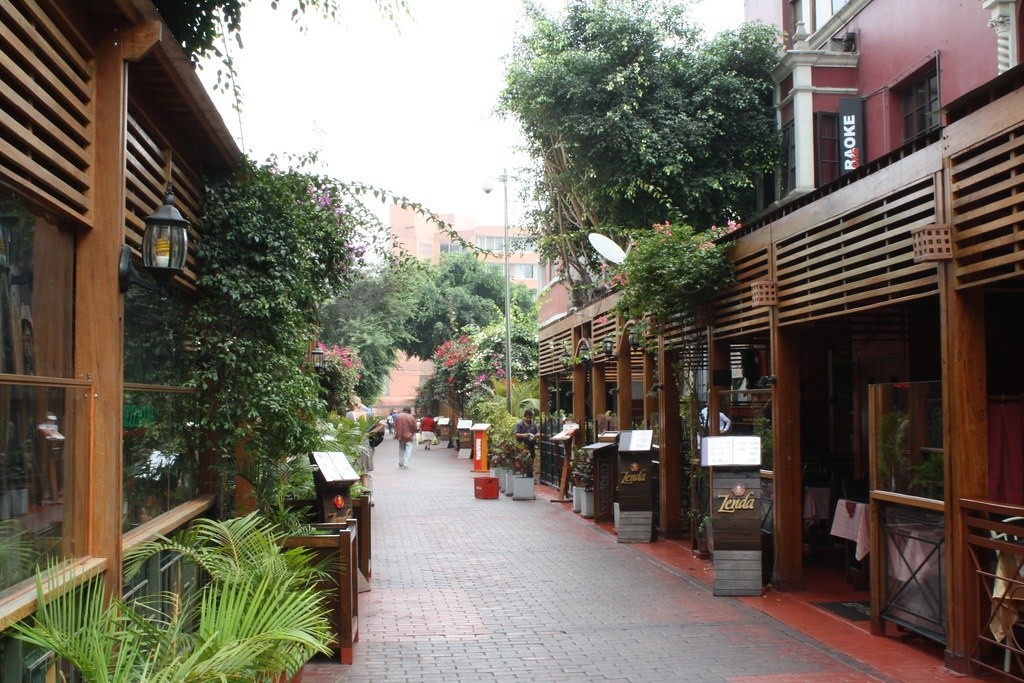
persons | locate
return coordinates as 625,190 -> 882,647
345,396 -> 435,505
515,410 -> 540,478
697,400 -> 732,435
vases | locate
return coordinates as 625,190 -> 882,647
572,486 -> 595,517
0,488 -> 28,519
493,466 -> 538,501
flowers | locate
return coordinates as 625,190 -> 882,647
570,445 -> 593,491
491,438 -> 533,476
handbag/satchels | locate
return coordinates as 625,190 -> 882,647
369,430 -> 385,447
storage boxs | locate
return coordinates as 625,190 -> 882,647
474,477 -> 499,499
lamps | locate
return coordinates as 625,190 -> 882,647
602,333 -> 618,362
118,180 -> 189,299
579,339 -> 594,368
312,342 -> 324,373
628,325 -> 646,356
562,349 -> 573,373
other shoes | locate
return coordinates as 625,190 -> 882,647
401,465 -> 408,469
425,445 -> 430,450
